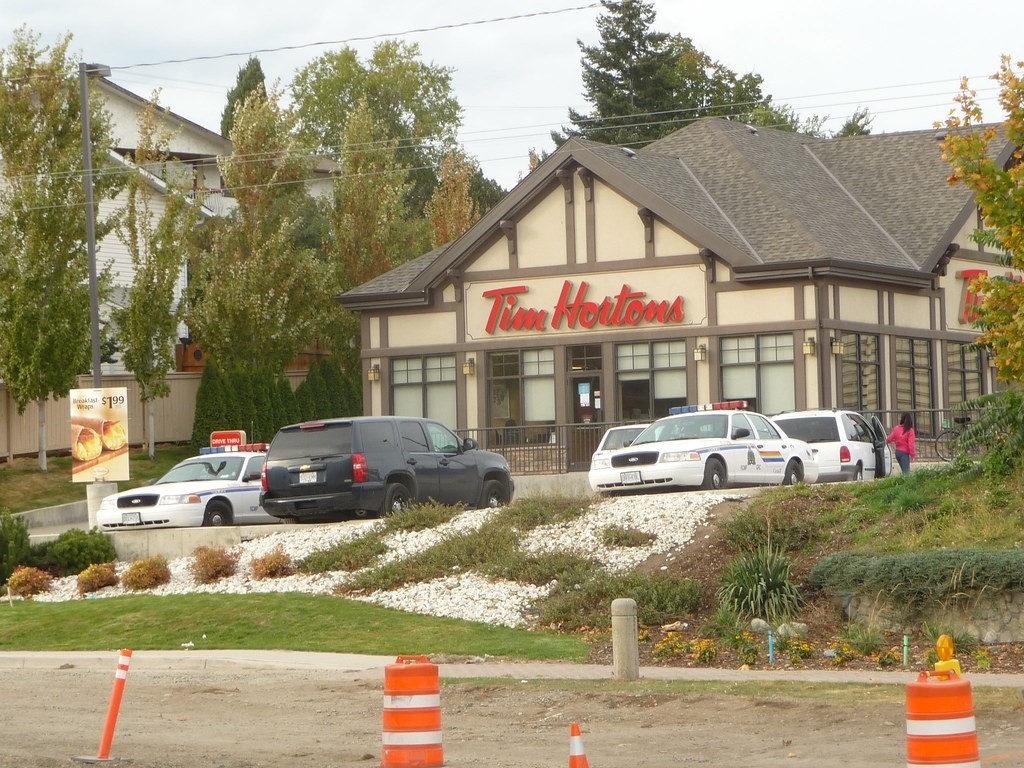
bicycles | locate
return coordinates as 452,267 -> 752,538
934,419 -> 1009,463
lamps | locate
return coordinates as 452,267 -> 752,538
829,336 -> 843,355
367,364 -> 380,381
693,344 -> 706,361
463,358 -> 474,375
802,337 -> 815,355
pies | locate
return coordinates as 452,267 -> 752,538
70,416 -> 126,462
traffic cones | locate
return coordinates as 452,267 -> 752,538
568,721 -> 590,768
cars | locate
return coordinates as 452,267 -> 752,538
97,443 -> 285,532
596,401 -> 819,492
589,424 -> 664,495
769,406 -> 893,483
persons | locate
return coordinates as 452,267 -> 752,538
884,412 -> 917,474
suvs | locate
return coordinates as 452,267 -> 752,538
258,415 -> 515,524
